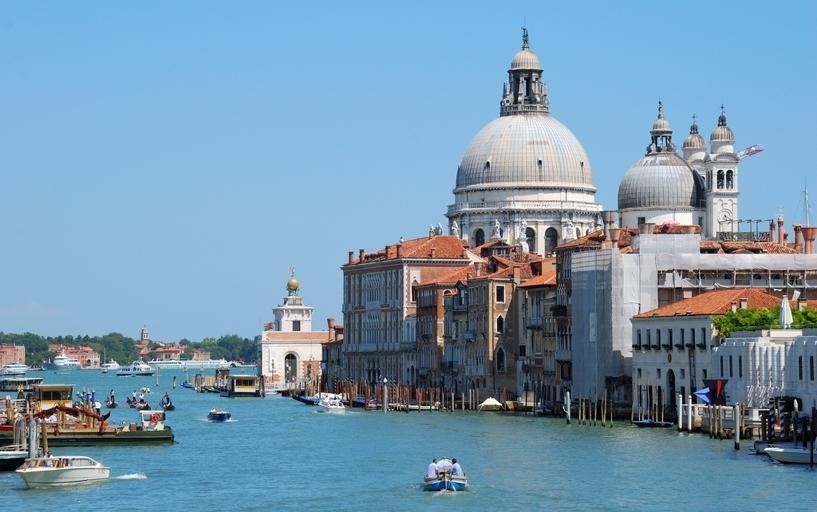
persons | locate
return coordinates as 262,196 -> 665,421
153,421 -> 164,431
110,389 -> 115,403
427,458 -> 438,478
162,392 -> 169,404
94,400 -> 101,416
106,400 -> 114,407
450,458 -> 464,481
43,450 -> 55,467
210,408 -> 216,412
36,413 -> 57,423
132,386 -> 150,404
325,396 -> 329,402
330,398 -> 339,406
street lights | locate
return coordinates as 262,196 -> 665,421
382,378 -> 387,413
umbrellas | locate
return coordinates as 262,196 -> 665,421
779,294 -> 793,329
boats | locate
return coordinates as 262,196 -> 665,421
182,382 -> 193,388
207,410 -> 230,422
0,384 -> 109,426
134,401 -> 152,411
126,396 -> 139,407
116,361 -> 154,376
293,395 -> 324,405
0,444 -> 46,467
633,419 -> 673,429
320,396 -> 345,410
763,447 -> 816,464
477,397 -> 502,411
17,454 -> 110,488
162,398 -> 176,412
101,368 -> 108,373
753,431 -> 817,453
0,364 -> 28,377
104,396 -> 119,408
365,399 -> 377,410
424,458 -> 468,492
0,376 -> 44,392
531,404 -> 552,413
102,358 -> 120,370
352,395 -> 366,407
